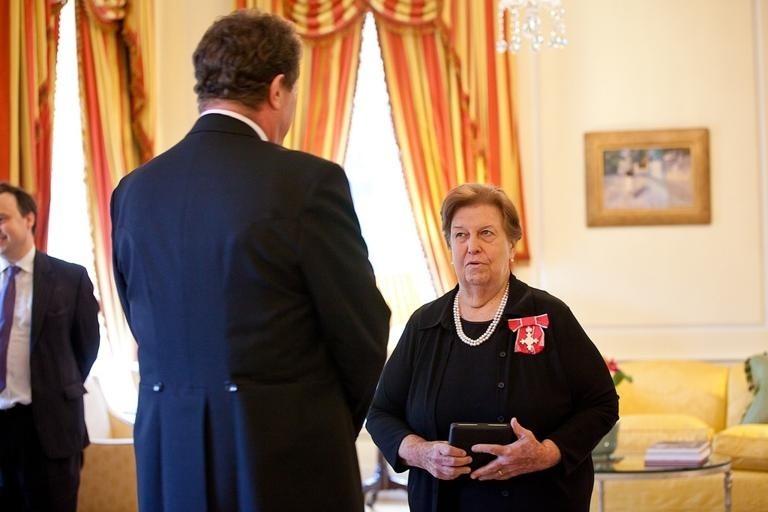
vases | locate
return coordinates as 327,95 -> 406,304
592,417 -> 621,457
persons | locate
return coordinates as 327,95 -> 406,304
366,184 -> 620,512
110,5 -> 392,512
0,182 -> 100,512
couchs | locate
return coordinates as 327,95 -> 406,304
590,356 -> 767,512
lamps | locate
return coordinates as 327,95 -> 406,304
493,0 -> 571,56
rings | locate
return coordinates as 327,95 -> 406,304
498,470 -> 504,475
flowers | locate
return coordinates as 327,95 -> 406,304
601,357 -> 635,391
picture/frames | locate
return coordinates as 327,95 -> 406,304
583,125 -> 713,227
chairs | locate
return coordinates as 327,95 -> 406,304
71,375 -> 145,512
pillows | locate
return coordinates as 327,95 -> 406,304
740,353 -> 766,425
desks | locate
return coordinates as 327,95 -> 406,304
591,447 -> 741,511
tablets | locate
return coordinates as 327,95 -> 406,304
449,423 -> 513,469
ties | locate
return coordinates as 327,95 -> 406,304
0,265 -> 21,392
